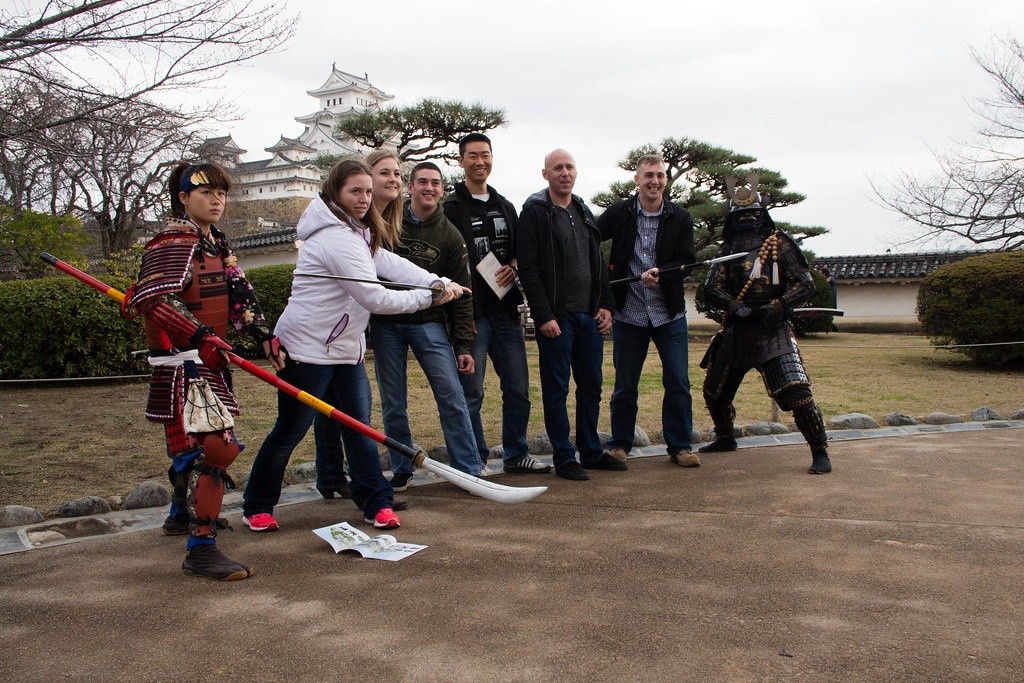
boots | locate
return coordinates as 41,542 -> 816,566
699,427 -> 737,452
808,449 -> 831,474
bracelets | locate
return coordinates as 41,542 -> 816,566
510,266 -> 518,271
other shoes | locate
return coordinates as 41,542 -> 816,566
182,540 -> 255,581
163,511 -> 227,535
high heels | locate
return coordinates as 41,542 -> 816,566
392,497 -> 408,511
317,480 -> 353,499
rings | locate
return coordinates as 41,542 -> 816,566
649,282 -> 652,285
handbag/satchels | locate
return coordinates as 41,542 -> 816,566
184,378 -> 234,434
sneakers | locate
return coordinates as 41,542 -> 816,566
389,471 -> 413,492
671,447 -> 700,467
478,466 -> 494,479
556,461 -> 593,480
586,453 -> 629,470
364,506 -> 399,529
504,445 -> 550,473
608,447 -> 627,465
242,512 -> 280,531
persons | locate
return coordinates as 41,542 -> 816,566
597,155 -> 700,468
441,133 -> 551,475
314,149 -> 407,507
366,162 -> 480,493
131,162 -> 286,580
695,173 -> 832,474
516,149 -> 627,480
242,159 -> 473,532
819,268 -> 840,332
